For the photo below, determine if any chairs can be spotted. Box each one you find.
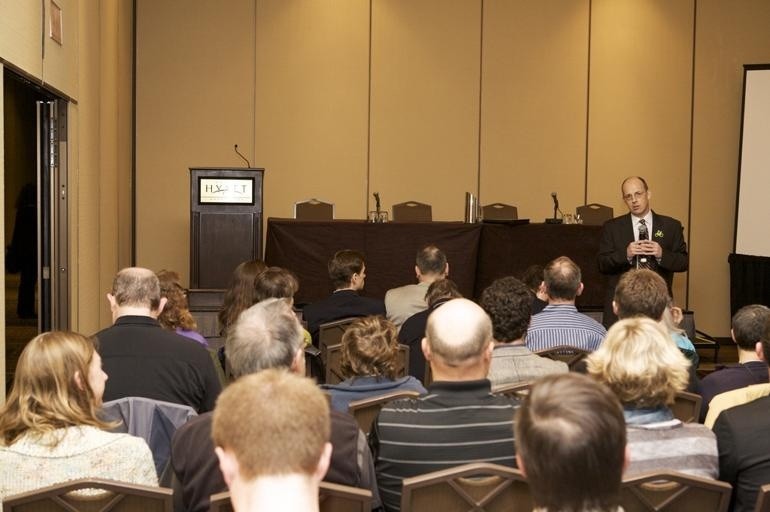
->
[349,390,422,440]
[619,470,732,510]
[319,482,372,512]
[532,345,591,365]
[301,317,432,390]
[210,491,232,510]
[491,381,535,400]
[481,201,519,220]
[292,196,334,219]
[401,461,533,509]
[8,478,175,512]
[752,484,770,511]
[392,199,434,220]
[670,391,703,422]
[574,202,613,224]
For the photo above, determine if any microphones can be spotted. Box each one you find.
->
[373,191,380,211]
[234,144,250,168]
[639,225,648,263]
[551,192,559,207]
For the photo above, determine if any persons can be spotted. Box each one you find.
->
[599,176,689,330]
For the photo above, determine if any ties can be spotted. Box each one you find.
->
[637,218,651,272]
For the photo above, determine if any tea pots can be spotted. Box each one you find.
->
[464,191,485,223]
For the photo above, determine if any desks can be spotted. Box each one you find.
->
[265,216,612,308]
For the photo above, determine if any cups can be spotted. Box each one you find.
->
[377,211,388,225]
[573,213,584,226]
[367,212,378,223]
[561,211,573,224]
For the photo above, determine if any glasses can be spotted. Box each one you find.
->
[623,190,644,199]
